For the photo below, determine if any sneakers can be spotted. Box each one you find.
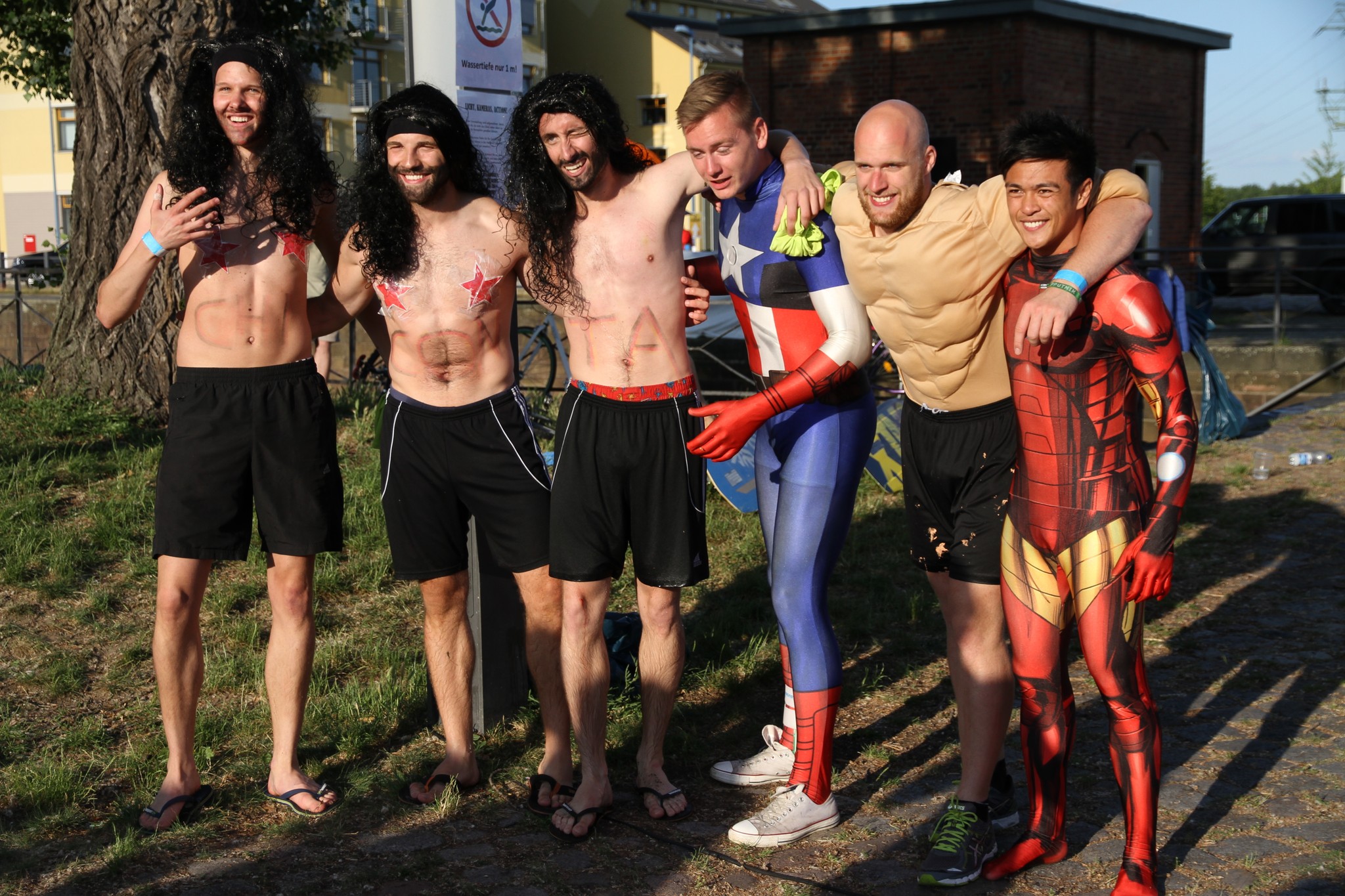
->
[913,788,997,885]
[927,757,1019,844]
[727,783,840,847]
[710,725,796,787]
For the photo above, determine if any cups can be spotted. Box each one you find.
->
[1253,452,1274,480]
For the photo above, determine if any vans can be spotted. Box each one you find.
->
[1198,194,1345,315]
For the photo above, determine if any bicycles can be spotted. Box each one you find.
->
[351,344,391,391]
[515,304,575,403]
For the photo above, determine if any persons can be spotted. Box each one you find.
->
[294,80,709,814]
[497,74,828,843]
[816,97,1152,888]
[96,38,390,835]
[673,68,880,846]
[995,116,1196,896]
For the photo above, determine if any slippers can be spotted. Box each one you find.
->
[397,773,482,805]
[139,784,214,836]
[263,781,343,817]
[548,802,614,843]
[527,773,576,815]
[638,786,693,821]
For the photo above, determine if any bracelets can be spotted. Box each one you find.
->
[1043,269,1088,305]
[141,231,167,257]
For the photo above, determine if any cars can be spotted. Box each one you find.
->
[11,241,74,287]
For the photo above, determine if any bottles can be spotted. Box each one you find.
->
[1289,450,1332,466]
